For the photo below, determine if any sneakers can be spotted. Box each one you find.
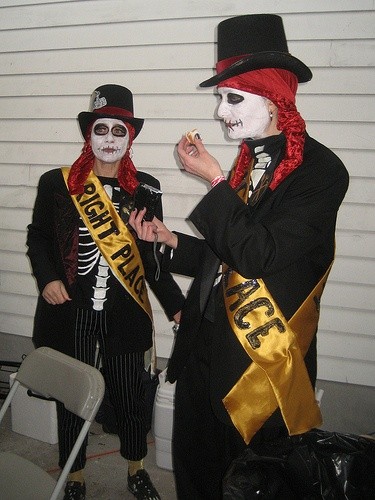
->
[126,467,160,500]
[62,480,86,500]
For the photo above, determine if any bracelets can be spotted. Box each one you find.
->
[171,321,180,333]
[210,175,227,188]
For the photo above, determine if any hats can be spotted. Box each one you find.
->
[199,14,313,87]
[78,84,145,141]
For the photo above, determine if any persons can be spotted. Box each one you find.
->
[128,13,350,500]
[24,85,188,500]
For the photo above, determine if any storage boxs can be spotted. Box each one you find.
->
[10,373,58,444]
[154,369,178,472]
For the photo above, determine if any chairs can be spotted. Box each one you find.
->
[0,347,106,500]
